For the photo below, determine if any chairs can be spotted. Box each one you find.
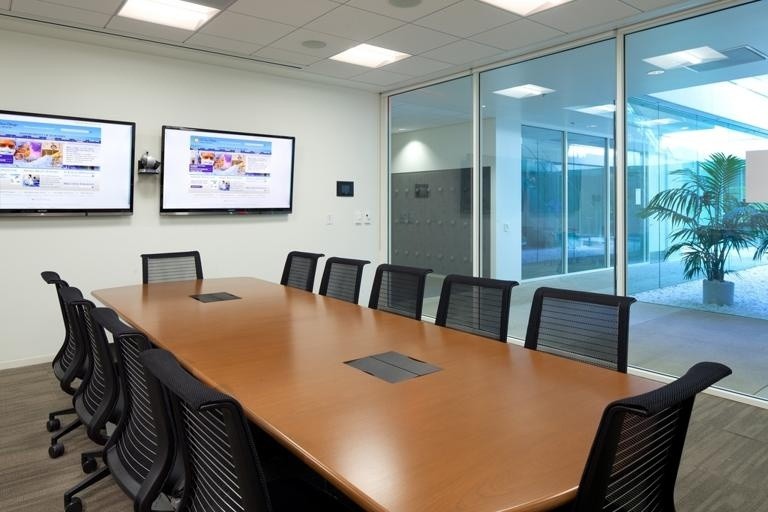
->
[524,287,637,374]
[140,249,206,284]
[62,309,186,512]
[321,256,370,304]
[129,350,275,512]
[281,249,323,293]
[57,285,111,471]
[369,263,432,321]
[33,271,88,458]
[431,276,520,340]
[574,360,735,511]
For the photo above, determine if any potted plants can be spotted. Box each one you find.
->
[640,152,767,306]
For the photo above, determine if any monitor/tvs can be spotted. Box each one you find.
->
[0,111,136,216]
[160,125,295,217]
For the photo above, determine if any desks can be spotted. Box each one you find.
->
[91,277,674,512]
